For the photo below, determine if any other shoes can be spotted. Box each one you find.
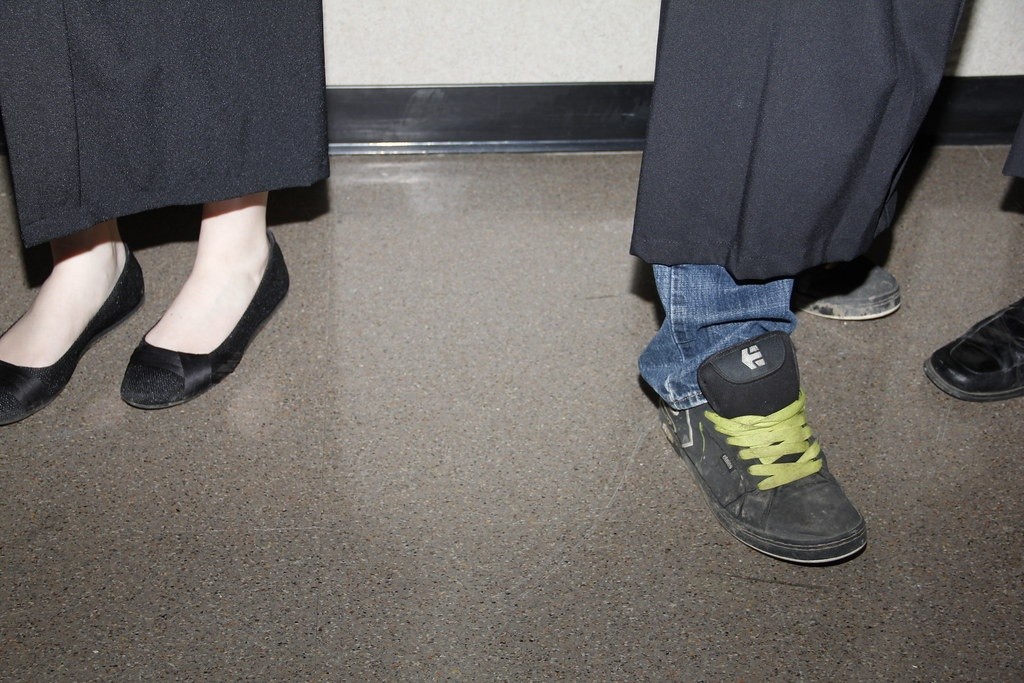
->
[0,242,145,423]
[121,230,288,408]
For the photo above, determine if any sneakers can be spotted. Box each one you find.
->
[792,255,901,319]
[660,331,868,564]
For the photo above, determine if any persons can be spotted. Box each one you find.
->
[625,1,971,566]
[0,0,331,429]
[920,293,1024,403]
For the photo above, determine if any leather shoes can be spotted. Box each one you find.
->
[924,294,1024,400]
